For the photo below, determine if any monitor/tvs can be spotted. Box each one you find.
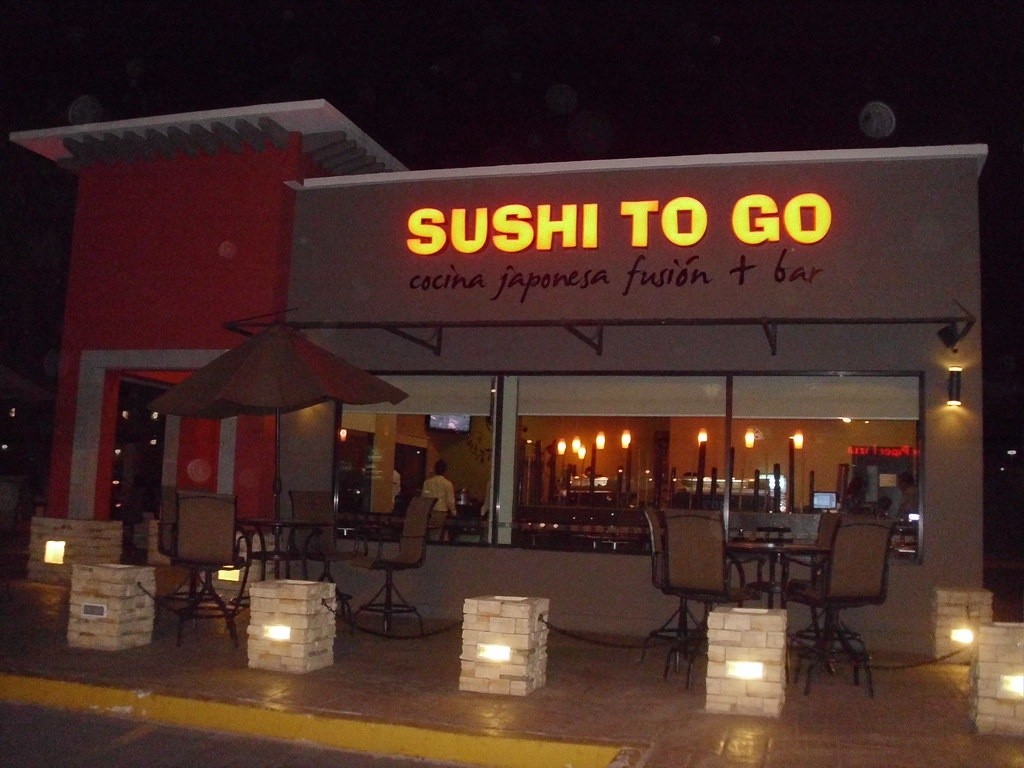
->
[425,415,472,434]
[813,492,837,509]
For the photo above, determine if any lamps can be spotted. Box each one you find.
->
[556,428,804,461]
[946,367,963,407]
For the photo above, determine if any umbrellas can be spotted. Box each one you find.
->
[145,321,408,521]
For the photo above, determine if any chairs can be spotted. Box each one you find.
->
[349,495,440,637]
[157,486,243,648]
[787,511,862,661]
[640,511,680,662]
[284,490,353,619]
[791,522,891,701]
[646,515,755,689]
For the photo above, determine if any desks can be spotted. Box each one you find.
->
[235,519,355,626]
[718,538,832,607]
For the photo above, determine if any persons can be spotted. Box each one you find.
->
[344,458,371,522]
[421,459,457,516]
[841,476,868,511]
[897,471,919,515]
